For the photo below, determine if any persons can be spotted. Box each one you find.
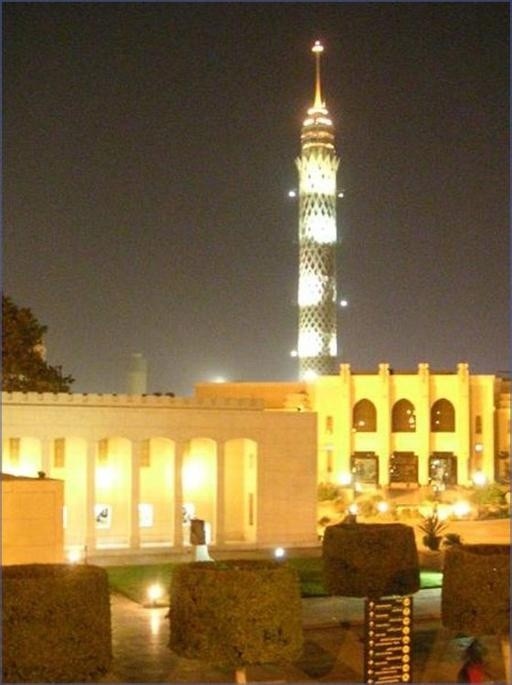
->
[456,640,496,685]
